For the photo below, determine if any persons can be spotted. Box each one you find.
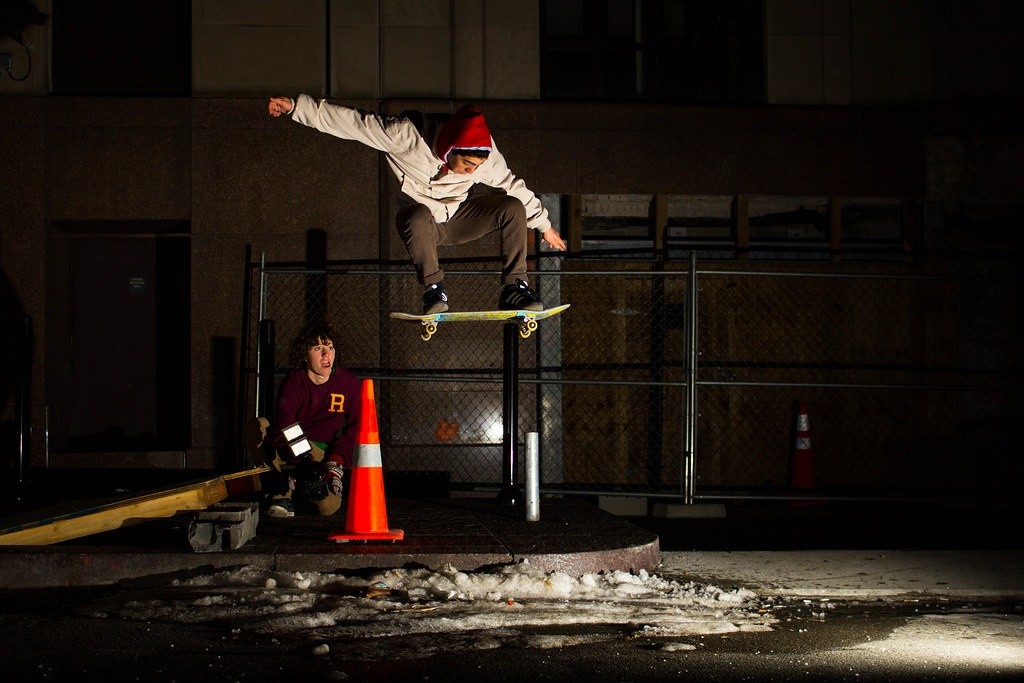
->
[244,321,361,518]
[266,92,568,317]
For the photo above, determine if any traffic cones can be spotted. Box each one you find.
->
[333,379,405,545]
[786,405,826,506]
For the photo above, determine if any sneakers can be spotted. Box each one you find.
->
[497,278,543,311]
[423,281,449,315]
[267,498,295,518]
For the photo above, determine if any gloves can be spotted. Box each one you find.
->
[273,473,297,499]
[323,461,344,495]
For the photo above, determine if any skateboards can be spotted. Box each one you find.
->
[388,301,572,342]
